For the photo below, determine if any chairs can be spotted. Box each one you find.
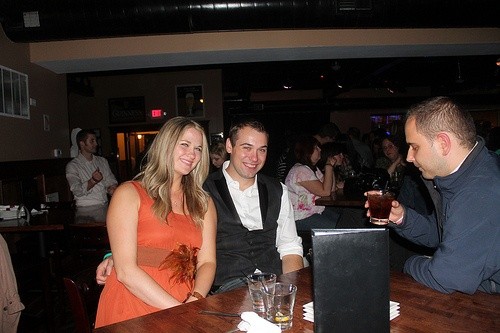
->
[61,265,105,333]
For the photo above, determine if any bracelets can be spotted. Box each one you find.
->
[394,211,404,225]
[92,176,98,182]
[104,253,112,260]
[326,163,333,167]
[187,292,205,301]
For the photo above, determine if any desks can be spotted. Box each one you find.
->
[316,188,396,206]
[93,263,500,333]
[0,204,108,333]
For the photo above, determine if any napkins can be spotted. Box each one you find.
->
[303,300,401,323]
[237,311,282,333]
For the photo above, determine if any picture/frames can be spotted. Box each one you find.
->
[174,84,205,119]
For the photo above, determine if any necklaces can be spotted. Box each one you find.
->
[171,191,183,206]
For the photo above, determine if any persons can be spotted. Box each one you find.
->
[209,132,230,169]
[65,130,119,207]
[364,96,500,295]
[134,139,153,173]
[259,121,435,255]
[93,116,218,328]
[97,119,303,296]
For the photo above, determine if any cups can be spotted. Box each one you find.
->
[261,283,297,330]
[367,190,395,226]
[247,273,277,311]
[48,148,61,158]
